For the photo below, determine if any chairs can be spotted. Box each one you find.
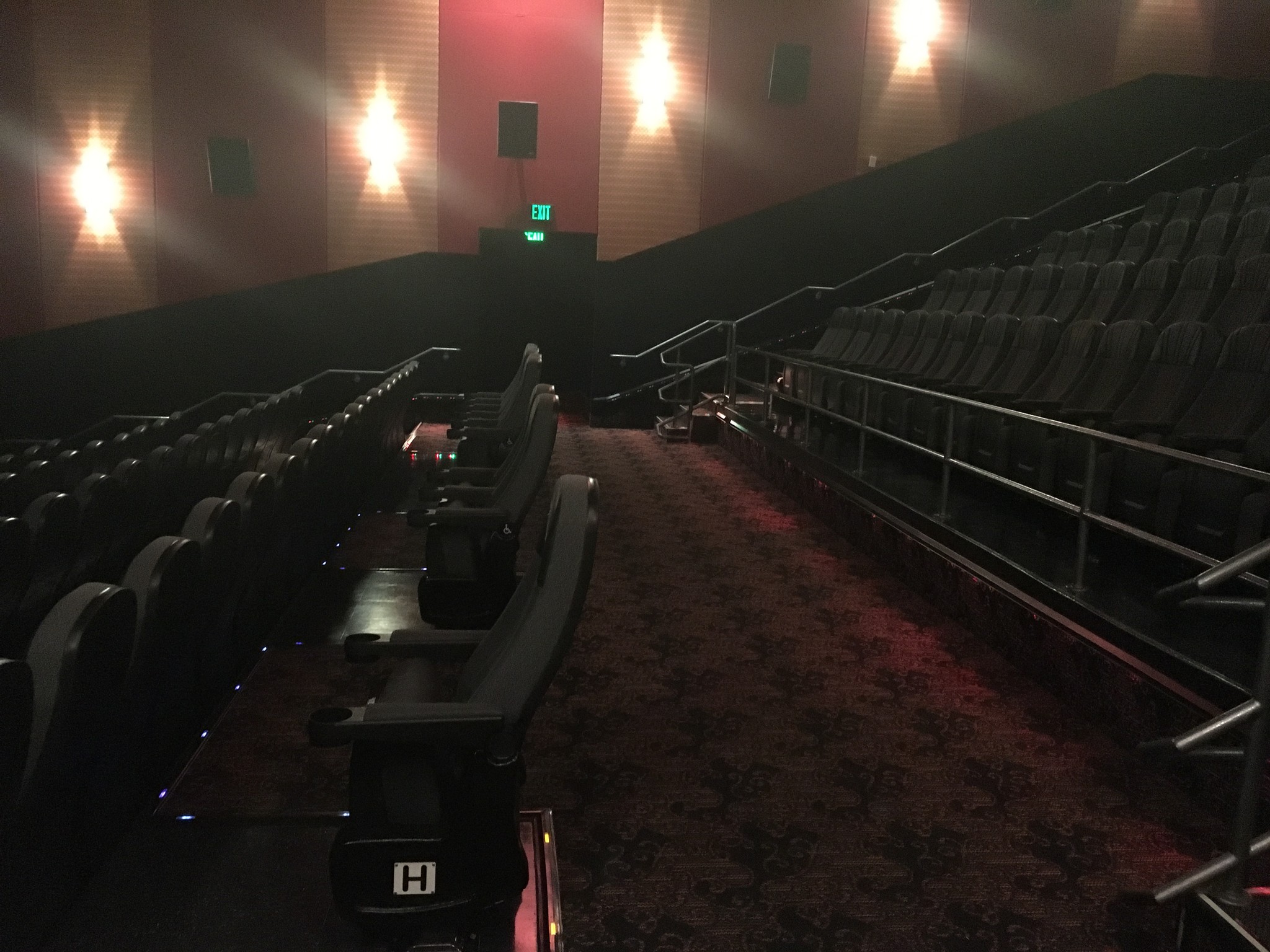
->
[0,342,598,952]
[777,153,1270,638]
